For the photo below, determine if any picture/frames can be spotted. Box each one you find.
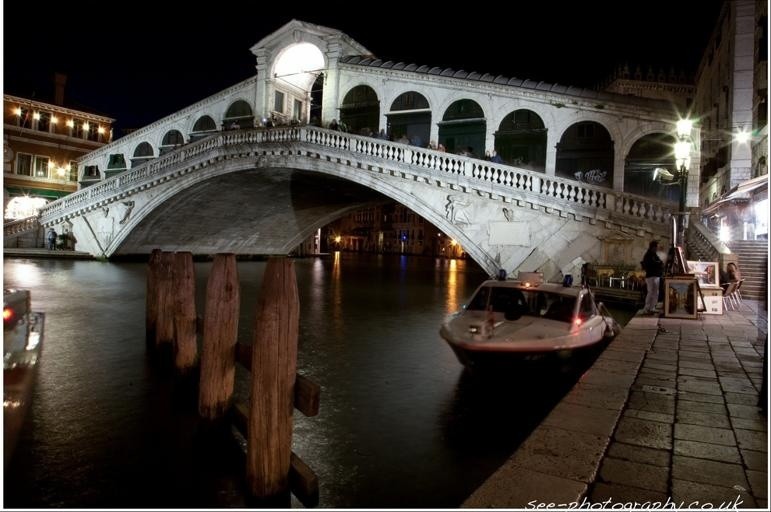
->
[662,276,697,321]
[685,259,721,287]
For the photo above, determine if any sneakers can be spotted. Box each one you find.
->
[641,307,660,316]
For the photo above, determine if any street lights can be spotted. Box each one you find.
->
[674,115,698,256]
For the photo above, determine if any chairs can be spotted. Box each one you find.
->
[720,278,745,312]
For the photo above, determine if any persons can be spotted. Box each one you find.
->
[368,127,504,165]
[641,240,666,316]
[337,120,348,133]
[230,115,307,131]
[46,227,71,251]
[328,118,339,129]
[117,199,136,224]
[720,262,740,294]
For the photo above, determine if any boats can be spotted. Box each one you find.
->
[2,284,50,460]
[437,267,623,375]
